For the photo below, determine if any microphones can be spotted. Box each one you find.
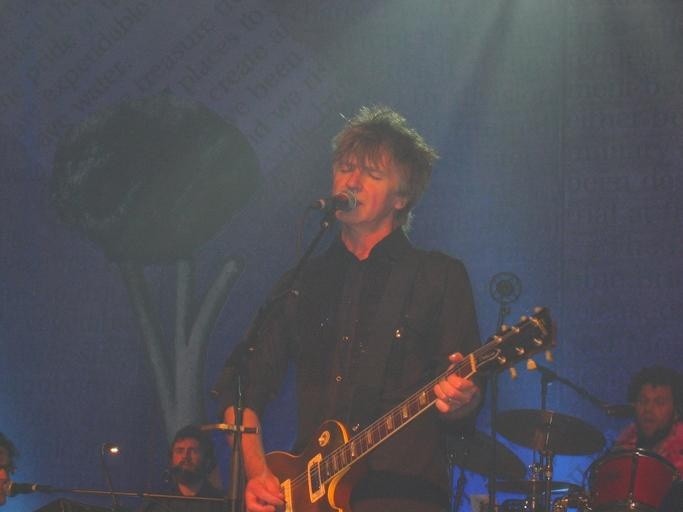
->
[490,273,522,304]
[310,190,356,212]
[3,481,50,497]
[605,404,636,416]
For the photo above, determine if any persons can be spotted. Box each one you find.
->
[588,364,683,512]
[0,432,20,506]
[143,425,230,512]
[218,105,489,511]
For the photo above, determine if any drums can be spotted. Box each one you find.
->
[585,449,677,510]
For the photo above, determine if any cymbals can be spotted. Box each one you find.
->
[482,478,572,491]
[493,411,608,456]
[454,429,528,480]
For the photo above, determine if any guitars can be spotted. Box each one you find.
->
[262,305,556,512]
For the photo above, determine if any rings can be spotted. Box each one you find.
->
[448,398,451,403]
